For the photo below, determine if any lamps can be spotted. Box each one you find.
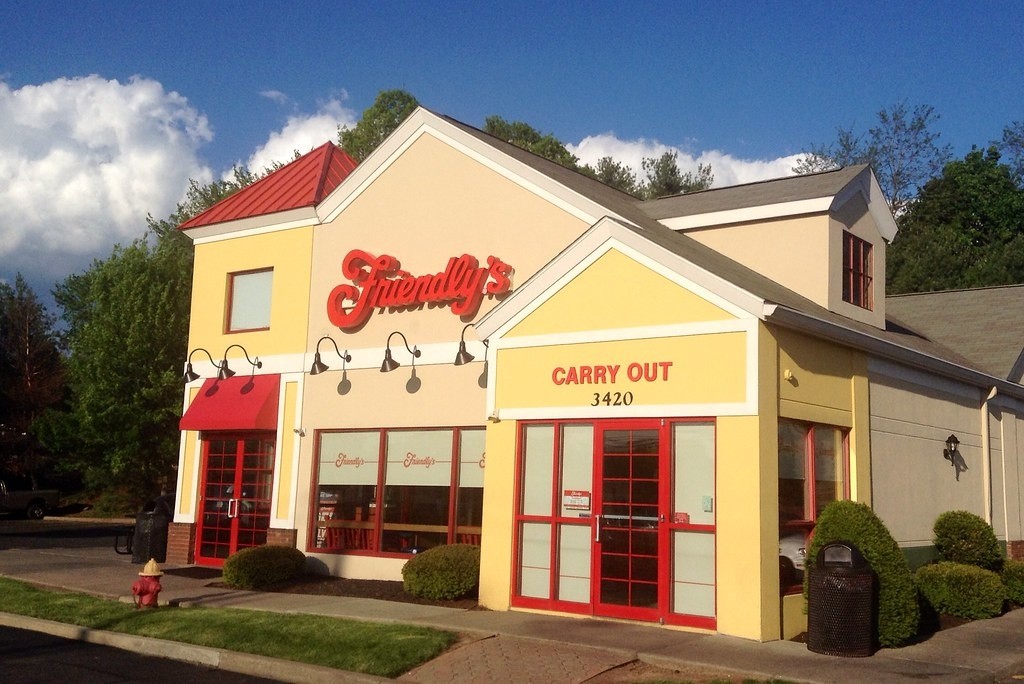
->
[182,348,222,383]
[454,323,489,366]
[943,434,960,466]
[219,344,262,380]
[380,331,421,372]
[309,337,352,375]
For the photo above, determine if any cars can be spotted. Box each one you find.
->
[779,512,806,592]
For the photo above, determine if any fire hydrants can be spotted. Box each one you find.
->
[133,558,164,608]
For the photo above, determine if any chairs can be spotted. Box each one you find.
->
[324,515,478,550]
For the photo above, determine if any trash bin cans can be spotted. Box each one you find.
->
[131,503,170,565]
[807,541,878,659]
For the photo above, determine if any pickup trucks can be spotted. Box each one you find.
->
[0,480,60,521]
